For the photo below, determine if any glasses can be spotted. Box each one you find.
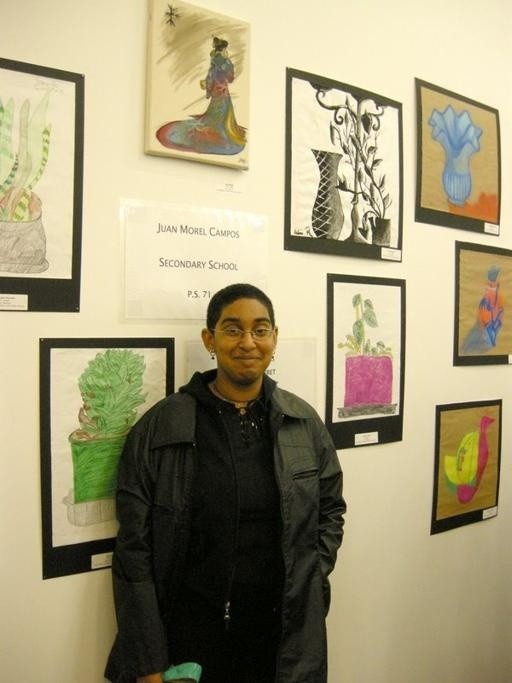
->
[209,328,272,341]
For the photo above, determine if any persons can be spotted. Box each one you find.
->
[104,285,347,683]
[156,37,247,156]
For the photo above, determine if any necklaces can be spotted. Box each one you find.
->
[212,383,257,415]
